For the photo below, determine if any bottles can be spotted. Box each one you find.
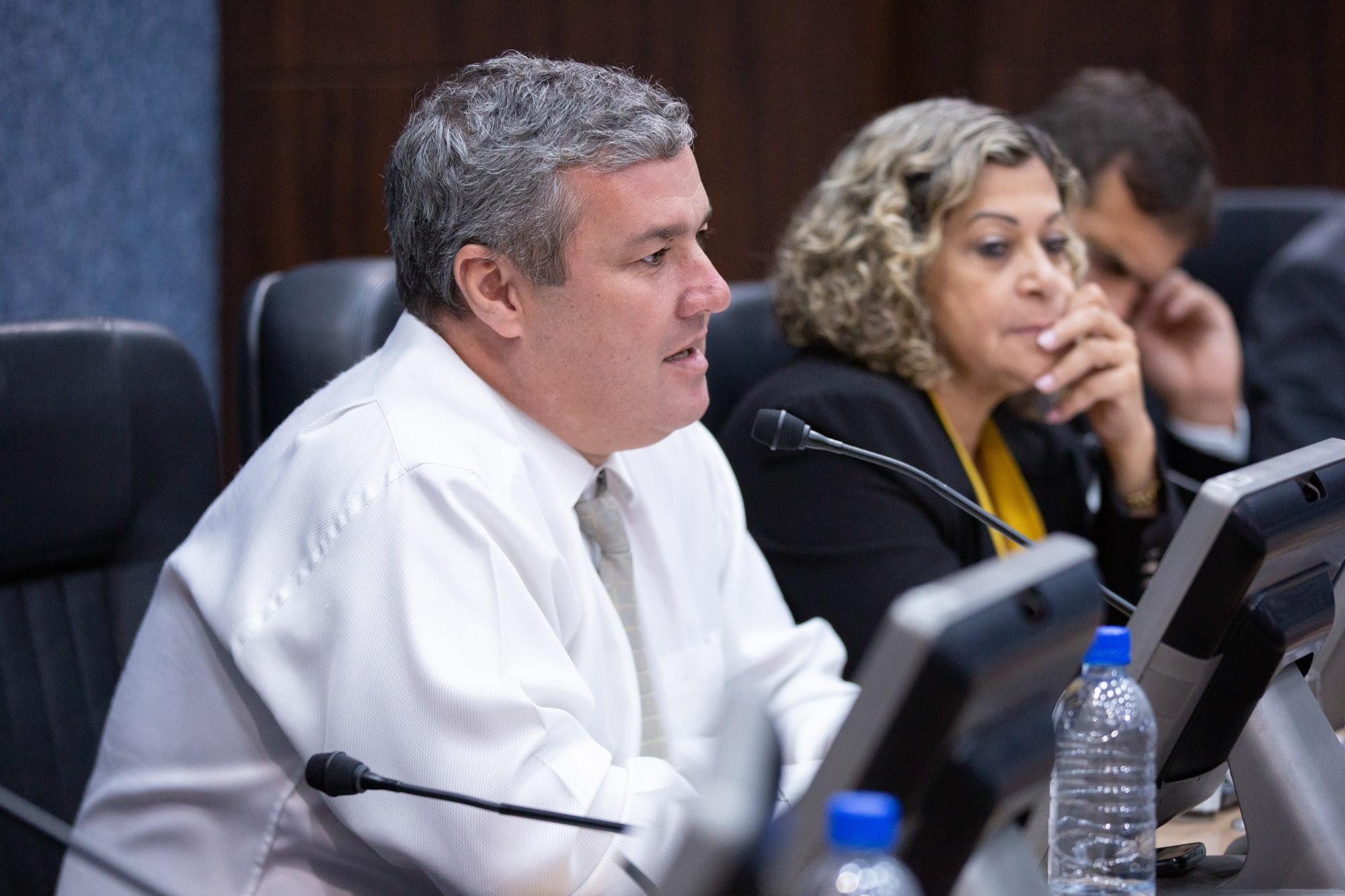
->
[794,789,926,896]
[1045,625,1160,896]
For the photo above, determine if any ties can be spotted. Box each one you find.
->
[574,469,666,759]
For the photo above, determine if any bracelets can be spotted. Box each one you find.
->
[1122,479,1157,509]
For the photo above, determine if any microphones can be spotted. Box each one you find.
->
[751,409,1136,616]
[304,753,635,837]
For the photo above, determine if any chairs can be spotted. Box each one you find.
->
[0,318,224,896]
[239,253,405,470]
[698,279,800,447]
[1173,185,1344,328]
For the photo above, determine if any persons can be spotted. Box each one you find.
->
[717,98,1182,683]
[57,53,862,896]
[992,69,1322,573]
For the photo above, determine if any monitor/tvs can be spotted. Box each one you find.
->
[767,438,1345,896]
